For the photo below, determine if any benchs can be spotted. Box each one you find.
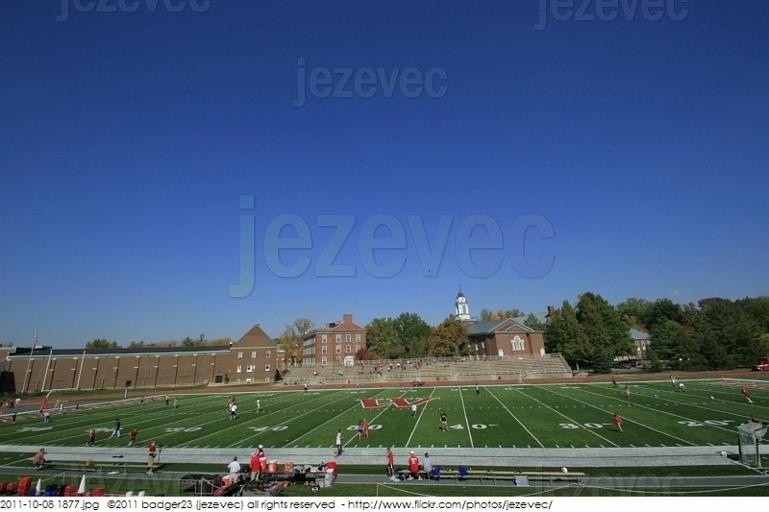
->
[397,469,586,488]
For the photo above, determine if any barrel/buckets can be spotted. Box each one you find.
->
[284,461,292,472]
[458,465,468,476]
[432,467,440,480]
[268,459,279,472]
[259,456,268,471]
[222,475,230,485]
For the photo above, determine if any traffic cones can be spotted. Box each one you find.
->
[33,478,42,495]
[76,474,86,494]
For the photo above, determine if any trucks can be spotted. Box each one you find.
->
[751,357,769,372]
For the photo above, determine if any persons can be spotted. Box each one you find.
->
[0,351,754,497]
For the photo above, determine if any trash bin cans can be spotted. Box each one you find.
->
[45,484,58,496]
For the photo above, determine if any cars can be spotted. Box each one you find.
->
[612,359,640,369]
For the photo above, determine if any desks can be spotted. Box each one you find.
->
[178,468,327,497]
